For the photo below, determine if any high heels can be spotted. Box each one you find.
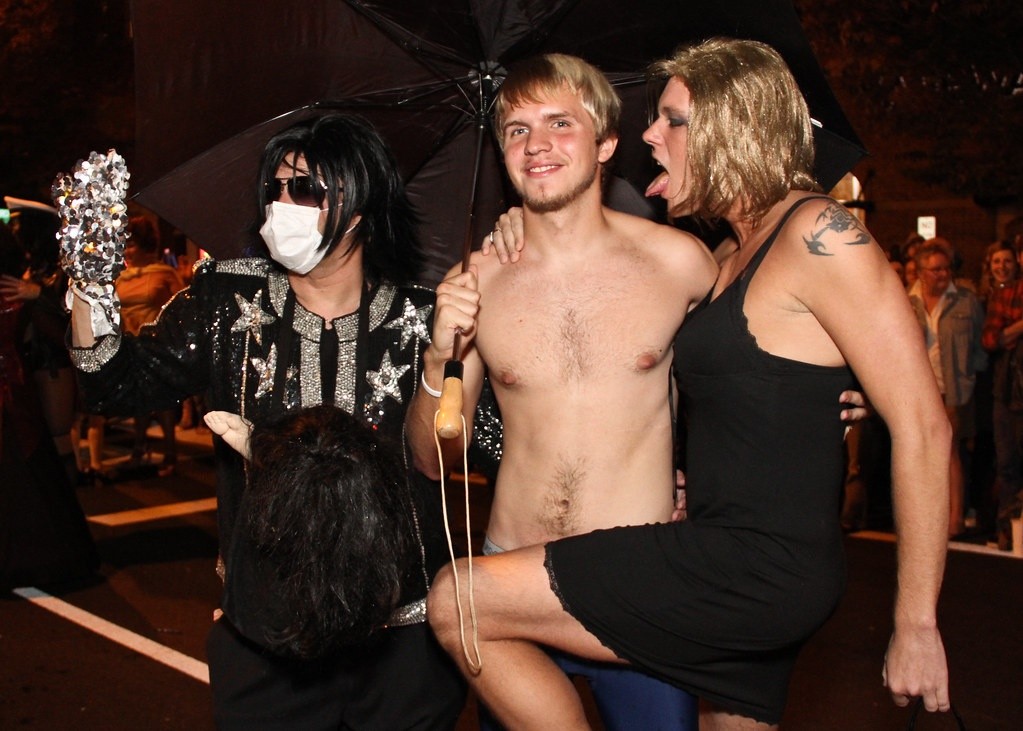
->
[156,455,178,476]
[127,439,152,466]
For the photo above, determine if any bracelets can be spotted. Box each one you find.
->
[421,371,442,398]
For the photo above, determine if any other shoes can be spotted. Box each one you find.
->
[999,532,1013,551]
[89,469,109,486]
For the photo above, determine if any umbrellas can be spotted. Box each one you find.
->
[128,1,846,434]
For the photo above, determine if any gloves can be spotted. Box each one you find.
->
[52,149,130,336]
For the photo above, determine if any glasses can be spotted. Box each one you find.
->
[261,177,344,207]
[920,266,950,274]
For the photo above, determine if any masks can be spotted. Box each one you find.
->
[260,200,348,276]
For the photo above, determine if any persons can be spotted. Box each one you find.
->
[3,201,213,483]
[422,39,956,731]
[54,117,438,731]
[407,54,874,731]
[853,215,1023,553]
